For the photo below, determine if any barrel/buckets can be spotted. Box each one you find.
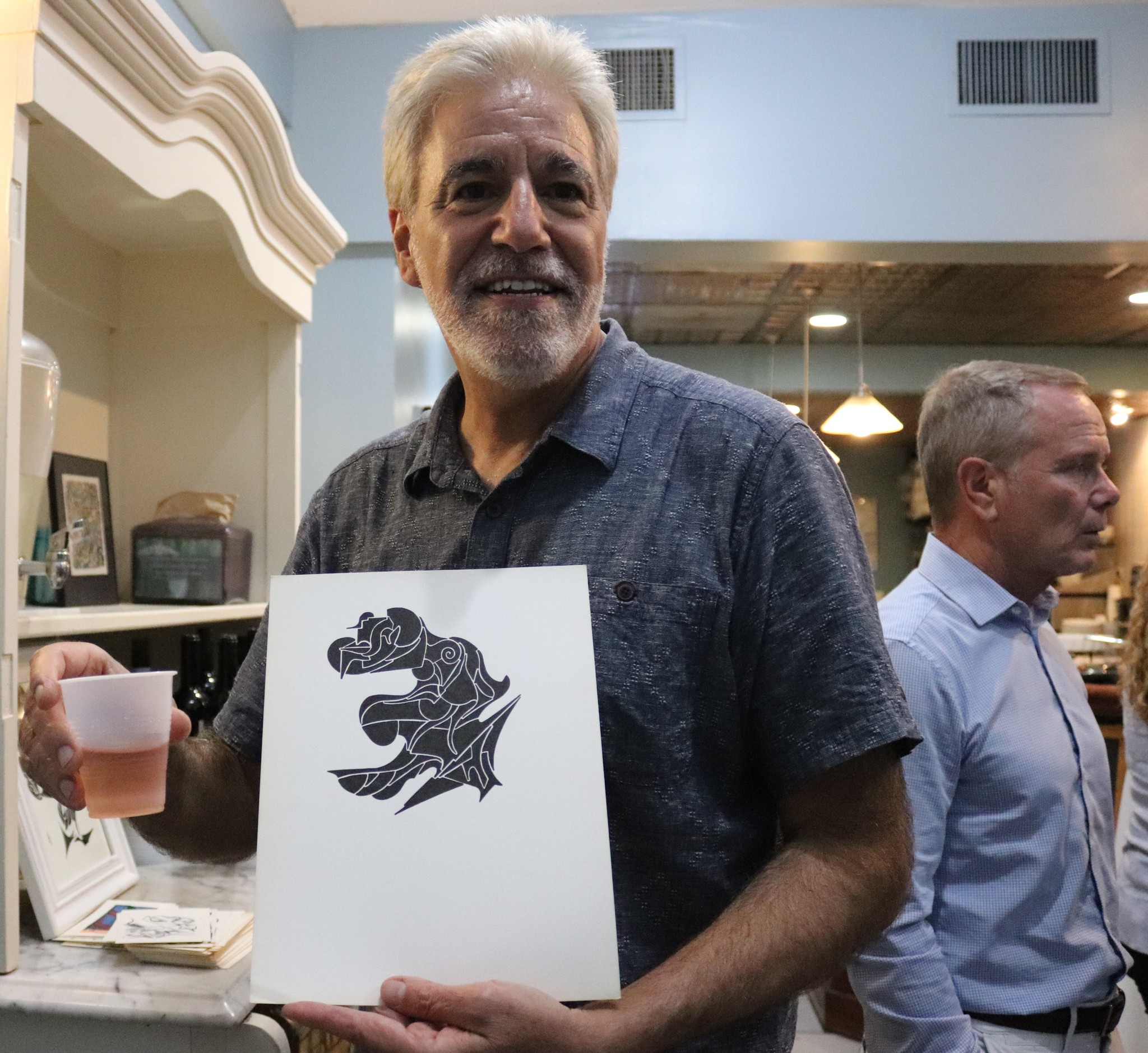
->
[18,328,84,608]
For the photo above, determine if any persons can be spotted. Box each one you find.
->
[19,18,925,1053]
[1113,563,1148,1015]
[844,361,1134,1053]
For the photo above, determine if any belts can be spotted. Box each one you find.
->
[962,982,1126,1034]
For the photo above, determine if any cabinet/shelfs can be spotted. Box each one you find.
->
[0,1,370,1053]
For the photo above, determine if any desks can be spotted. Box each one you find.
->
[1071,644,1132,828]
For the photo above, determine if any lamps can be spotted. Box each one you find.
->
[772,259,911,470]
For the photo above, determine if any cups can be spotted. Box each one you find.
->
[57,670,178,820]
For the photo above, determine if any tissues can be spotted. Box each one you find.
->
[125,487,256,606]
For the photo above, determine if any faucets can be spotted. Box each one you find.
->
[14,514,85,591]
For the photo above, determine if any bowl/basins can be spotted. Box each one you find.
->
[1080,664,1119,685]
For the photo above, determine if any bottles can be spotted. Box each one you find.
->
[32,527,59,605]
[129,627,257,738]
